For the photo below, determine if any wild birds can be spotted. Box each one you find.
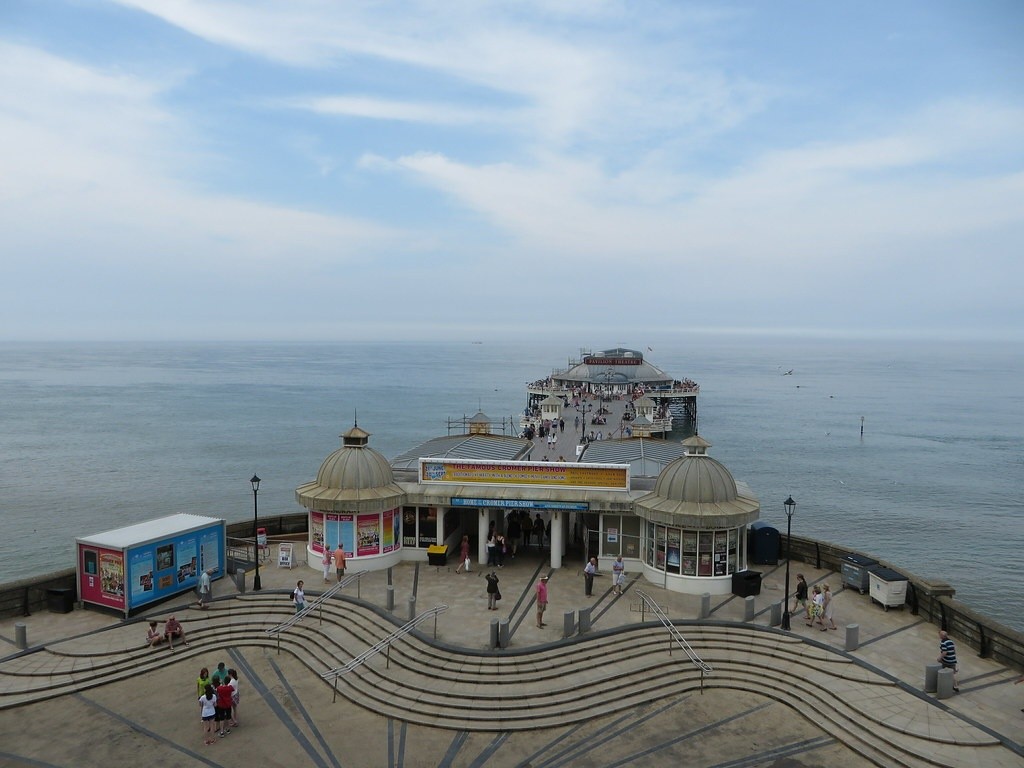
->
[824,431,831,437]
[783,368,794,375]
[648,345,653,351]
[493,388,498,391]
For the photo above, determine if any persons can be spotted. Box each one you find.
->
[592,413,606,425]
[322,544,347,585]
[524,401,564,449]
[620,406,637,437]
[197,662,239,745]
[613,555,624,594]
[164,615,189,651]
[584,558,596,598]
[542,456,549,461]
[456,536,473,574]
[789,574,837,631]
[293,580,305,621]
[197,569,213,609]
[485,573,501,610]
[574,416,586,434]
[487,527,505,569]
[654,405,669,421]
[489,508,560,558]
[556,456,566,462]
[528,377,697,407]
[590,430,613,441]
[937,631,960,692]
[536,576,549,629]
[146,620,163,648]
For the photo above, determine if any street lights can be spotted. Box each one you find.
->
[250,471,262,591]
[779,494,798,630]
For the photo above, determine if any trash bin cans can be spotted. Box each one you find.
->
[47,588,73,612]
[732,570,761,597]
[868,568,908,612]
[841,554,879,595]
[428,545,448,565]
[257,528,266,548]
[749,521,781,565]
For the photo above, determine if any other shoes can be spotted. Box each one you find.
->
[952,686,959,693]
[537,624,542,628]
[620,591,622,594]
[587,594,590,598]
[456,570,460,574]
[204,739,208,745]
[171,647,174,651]
[803,616,836,631]
[211,738,215,745]
[224,728,230,733]
[184,641,189,646]
[217,731,224,737]
[539,622,547,627]
[614,591,617,595]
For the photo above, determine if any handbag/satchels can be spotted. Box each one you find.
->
[465,557,471,571]
[617,570,626,585]
[494,590,501,600]
[290,590,296,599]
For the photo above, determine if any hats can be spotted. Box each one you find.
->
[539,575,548,579]
